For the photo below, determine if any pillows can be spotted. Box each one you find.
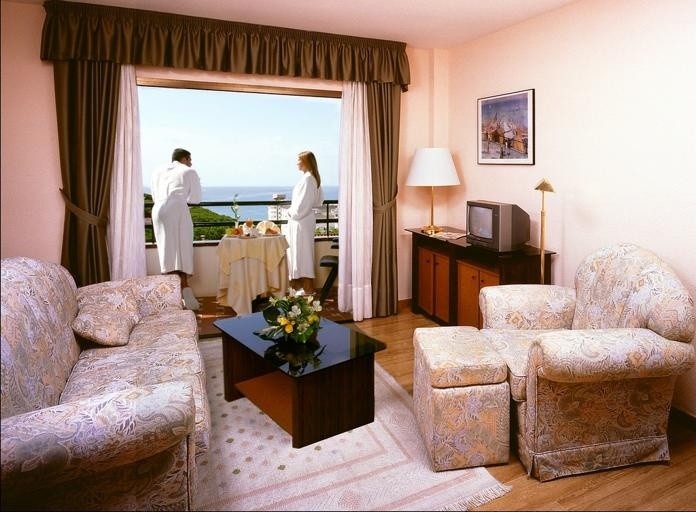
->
[70,283,142,347]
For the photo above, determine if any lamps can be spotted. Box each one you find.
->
[404,147,461,235]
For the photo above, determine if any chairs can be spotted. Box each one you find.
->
[477,241,696,482]
[319,237,339,306]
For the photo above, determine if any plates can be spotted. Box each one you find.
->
[226,231,281,239]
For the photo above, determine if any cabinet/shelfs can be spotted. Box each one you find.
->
[411,232,551,331]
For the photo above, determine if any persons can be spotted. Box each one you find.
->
[151,148,202,311]
[283,150,324,295]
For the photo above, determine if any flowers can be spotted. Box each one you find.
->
[230,193,241,222]
[250,286,324,343]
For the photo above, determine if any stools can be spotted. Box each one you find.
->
[412,325,511,473]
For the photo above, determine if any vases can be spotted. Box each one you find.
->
[307,331,318,344]
[234,221,240,229]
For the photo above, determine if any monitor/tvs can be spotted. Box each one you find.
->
[467,200,529,253]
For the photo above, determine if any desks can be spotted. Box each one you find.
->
[216,233,291,316]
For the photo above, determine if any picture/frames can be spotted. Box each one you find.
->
[476,88,535,165]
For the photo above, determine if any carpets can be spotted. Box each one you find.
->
[184,285,355,336]
[194,333,513,512]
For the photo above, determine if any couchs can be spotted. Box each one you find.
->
[0,245,211,512]
